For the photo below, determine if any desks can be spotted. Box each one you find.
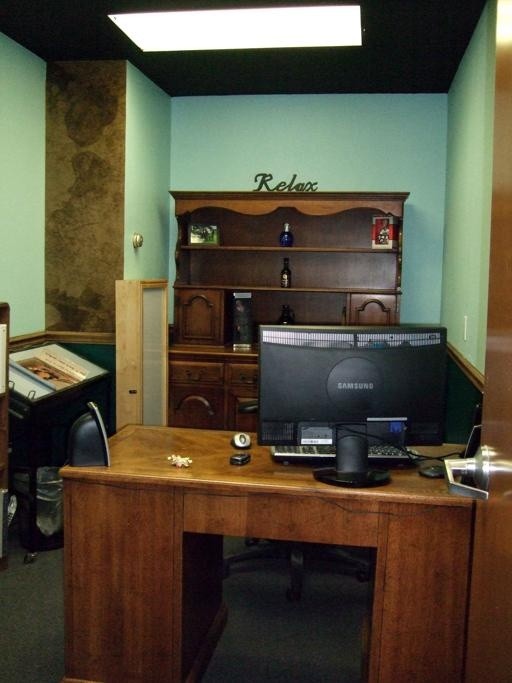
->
[60,425,477,683]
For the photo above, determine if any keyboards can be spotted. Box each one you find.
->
[270,442,410,465]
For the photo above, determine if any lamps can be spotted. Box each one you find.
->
[29,402,110,492]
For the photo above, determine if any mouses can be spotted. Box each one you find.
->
[232,433,251,449]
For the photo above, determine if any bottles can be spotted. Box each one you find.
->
[279,223,294,247]
[278,303,295,324]
[280,257,291,288]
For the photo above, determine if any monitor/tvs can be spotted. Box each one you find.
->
[259,324,447,488]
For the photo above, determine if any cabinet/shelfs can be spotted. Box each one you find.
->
[169,191,410,432]
